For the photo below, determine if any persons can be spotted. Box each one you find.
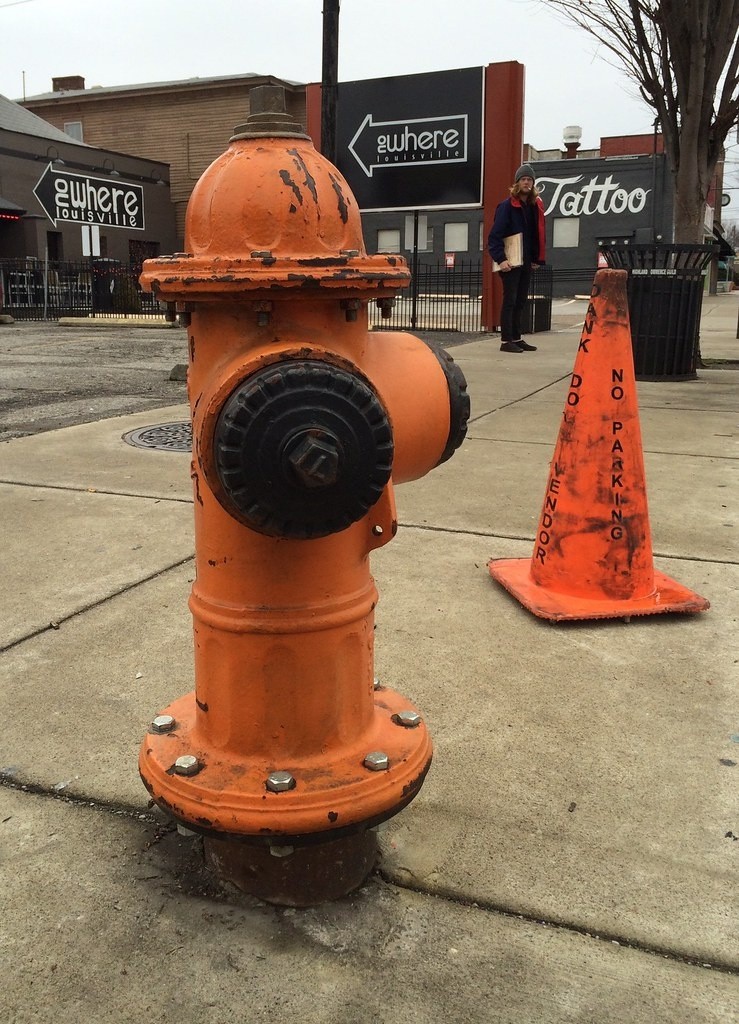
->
[487,164,547,353]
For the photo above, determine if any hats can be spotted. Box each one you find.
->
[515,165,535,185]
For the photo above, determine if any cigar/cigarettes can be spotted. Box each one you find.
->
[528,188,531,190]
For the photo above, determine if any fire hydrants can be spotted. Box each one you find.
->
[136,82,471,906]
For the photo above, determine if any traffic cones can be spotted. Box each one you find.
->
[487,267,711,628]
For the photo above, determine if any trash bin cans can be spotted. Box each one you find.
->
[598,242,719,383]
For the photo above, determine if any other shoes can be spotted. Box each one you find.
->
[513,340,537,351]
[500,341,523,353]
